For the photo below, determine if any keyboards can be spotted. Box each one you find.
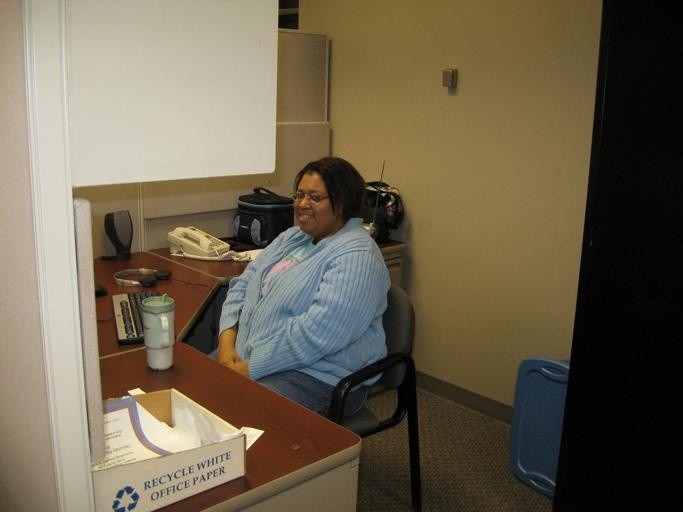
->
[112,290,176,345]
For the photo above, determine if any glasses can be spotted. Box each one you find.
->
[290,191,329,203]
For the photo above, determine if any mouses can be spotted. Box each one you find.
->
[95,285,108,297]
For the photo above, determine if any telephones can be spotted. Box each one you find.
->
[167,226,231,261]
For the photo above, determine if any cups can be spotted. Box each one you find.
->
[142,296,175,370]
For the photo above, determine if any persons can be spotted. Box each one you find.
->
[204,156,393,420]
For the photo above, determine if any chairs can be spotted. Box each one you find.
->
[324,282,422,512]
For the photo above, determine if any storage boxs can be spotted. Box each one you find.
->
[509,356,570,498]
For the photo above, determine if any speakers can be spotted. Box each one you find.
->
[100,210,133,261]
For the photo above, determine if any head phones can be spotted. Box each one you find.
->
[112,267,171,287]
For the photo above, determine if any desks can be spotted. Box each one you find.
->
[92,236,406,512]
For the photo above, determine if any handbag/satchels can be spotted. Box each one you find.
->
[233,186,294,249]
